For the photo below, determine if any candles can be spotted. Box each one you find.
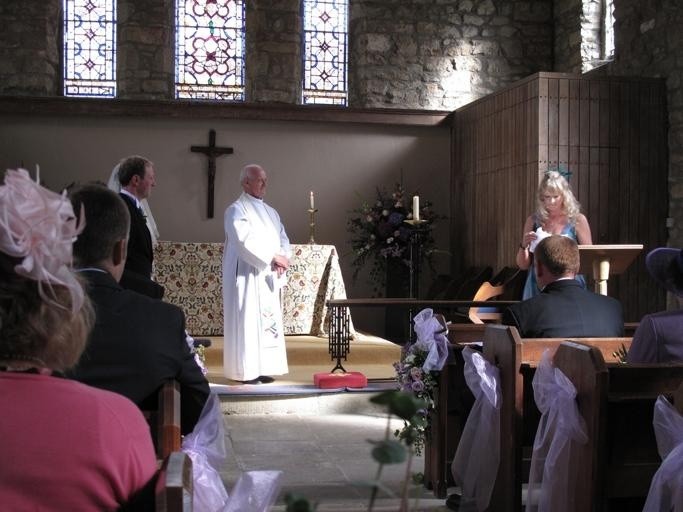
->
[310,191,314,210]
[413,195,420,220]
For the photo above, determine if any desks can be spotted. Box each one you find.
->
[153,241,356,336]
[325,298,523,373]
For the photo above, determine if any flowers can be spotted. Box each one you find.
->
[393,347,437,453]
[343,174,445,298]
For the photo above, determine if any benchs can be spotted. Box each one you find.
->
[424,322,682,512]
[139,382,195,512]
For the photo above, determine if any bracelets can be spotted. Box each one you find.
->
[520,243,526,250]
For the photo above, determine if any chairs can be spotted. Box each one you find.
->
[426,264,491,321]
[453,267,526,323]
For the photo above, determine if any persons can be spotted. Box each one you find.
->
[219,161,294,385]
[113,158,165,303]
[1,160,172,512]
[605,229,683,462]
[514,168,593,305]
[19,184,210,462]
[501,233,625,455]
[107,155,163,284]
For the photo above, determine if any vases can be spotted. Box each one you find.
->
[385,247,418,342]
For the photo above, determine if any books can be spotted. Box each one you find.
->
[266,269,289,292]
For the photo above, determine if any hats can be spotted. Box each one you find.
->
[646,248,681,299]
